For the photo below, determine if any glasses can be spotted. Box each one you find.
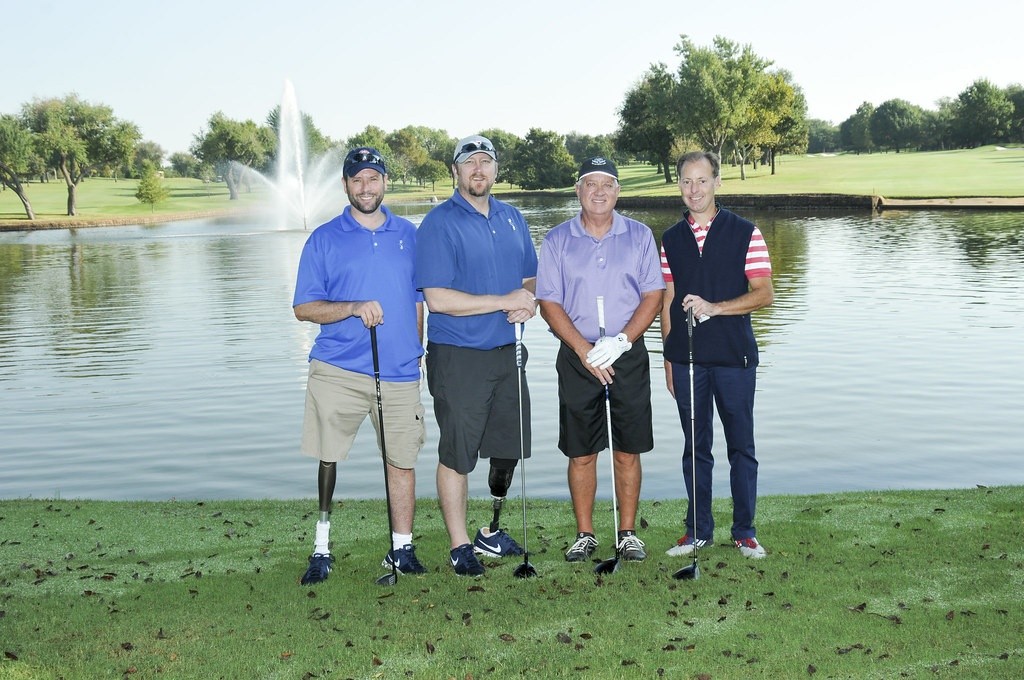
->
[454,141,497,164]
[344,152,385,174]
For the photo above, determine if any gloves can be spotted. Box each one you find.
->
[586,333,632,370]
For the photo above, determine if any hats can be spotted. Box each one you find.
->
[578,156,619,182]
[454,135,496,163]
[343,147,386,177]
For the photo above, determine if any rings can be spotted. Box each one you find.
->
[533,298,536,300]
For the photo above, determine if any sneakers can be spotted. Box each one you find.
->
[474,527,523,558]
[450,542,485,578]
[381,544,428,576]
[301,553,335,586]
[665,535,714,557]
[732,537,766,560]
[618,535,646,562]
[565,531,598,562]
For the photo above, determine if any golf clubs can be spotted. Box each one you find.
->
[512,322,538,578]
[594,294,623,575]
[369,325,399,587]
[669,300,702,580]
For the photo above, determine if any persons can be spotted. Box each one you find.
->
[534,156,667,563]
[292,148,424,588]
[660,152,775,560]
[414,134,540,578]
